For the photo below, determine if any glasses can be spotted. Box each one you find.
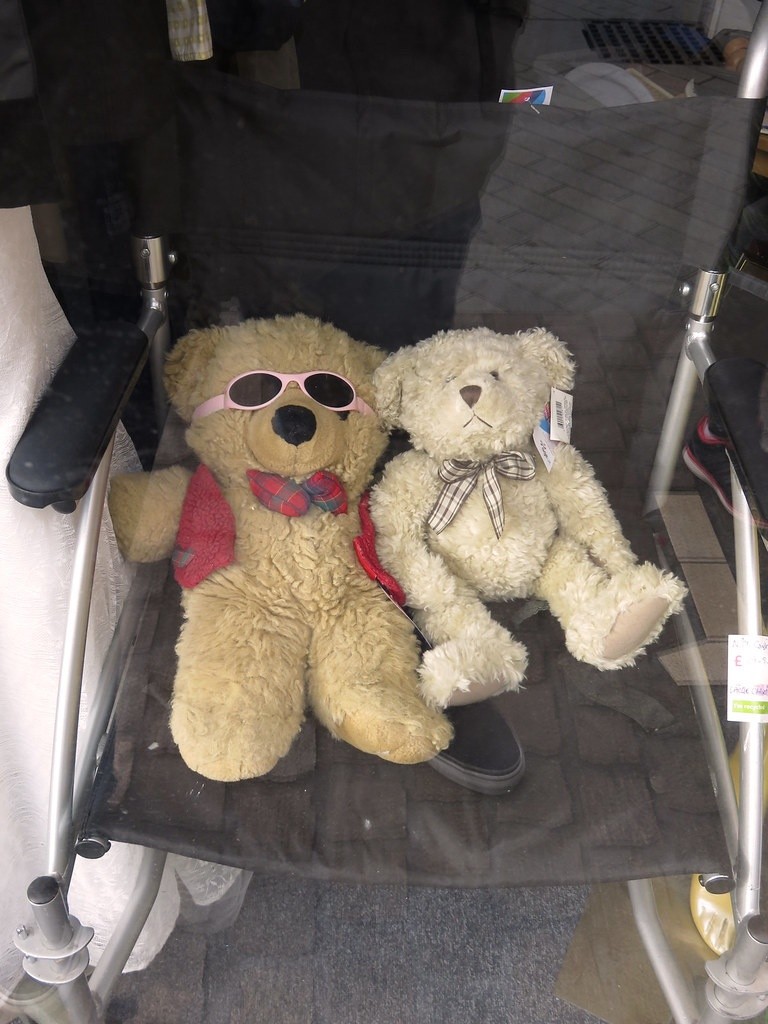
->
[189,371,376,423]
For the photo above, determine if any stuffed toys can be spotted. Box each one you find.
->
[368,325,691,712]
[109,310,458,784]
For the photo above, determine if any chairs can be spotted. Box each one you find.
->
[0,0,768,1024]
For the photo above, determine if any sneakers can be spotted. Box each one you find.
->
[427,699,524,794]
[684,417,768,536]
